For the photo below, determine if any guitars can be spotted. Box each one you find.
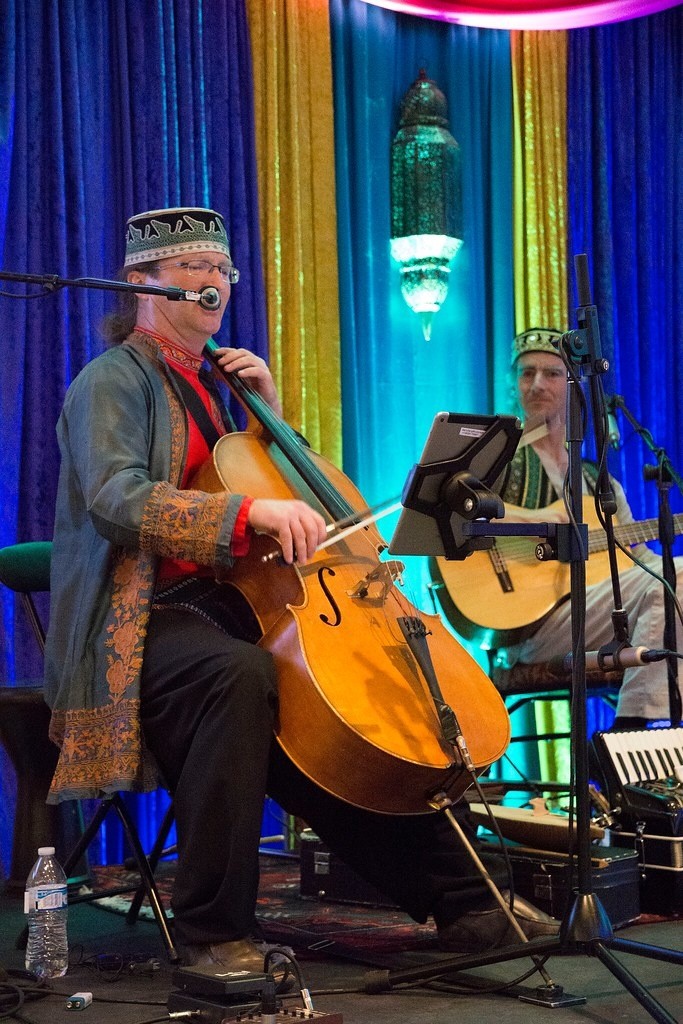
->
[428,492,683,651]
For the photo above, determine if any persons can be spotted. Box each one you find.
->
[40,205,575,984]
[471,328,682,805]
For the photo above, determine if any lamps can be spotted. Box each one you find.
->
[388,67,465,342]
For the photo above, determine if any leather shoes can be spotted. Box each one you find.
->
[172,939,296,995]
[433,890,563,954]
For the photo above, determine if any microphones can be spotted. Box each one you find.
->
[601,389,621,449]
[562,645,672,676]
[200,286,221,310]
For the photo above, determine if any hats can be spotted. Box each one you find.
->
[511,327,564,367]
[124,207,231,266]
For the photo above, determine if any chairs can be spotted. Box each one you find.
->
[424,555,623,812]
[1,538,183,967]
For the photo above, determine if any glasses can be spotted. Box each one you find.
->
[156,261,241,284]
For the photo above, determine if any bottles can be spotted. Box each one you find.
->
[24,847,69,979]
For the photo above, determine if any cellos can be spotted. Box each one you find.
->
[195,334,563,994]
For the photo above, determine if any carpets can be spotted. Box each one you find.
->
[90,850,443,963]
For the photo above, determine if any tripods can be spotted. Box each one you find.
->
[351,253,683,1023]
